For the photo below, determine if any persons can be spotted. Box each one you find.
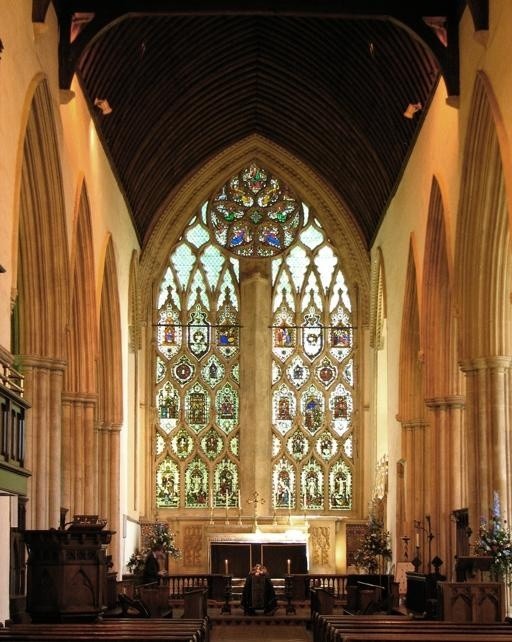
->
[140,542,168,589]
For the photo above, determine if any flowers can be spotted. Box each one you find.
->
[141,523,183,560]
[352,513,394,568]
[475,492,511,582]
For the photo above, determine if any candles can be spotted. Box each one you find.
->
[288,559,291,576]
[225,559,229,575]
[415,534,420,546]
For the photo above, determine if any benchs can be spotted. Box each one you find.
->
[311,611,512,642]
[0,616,211,642]
